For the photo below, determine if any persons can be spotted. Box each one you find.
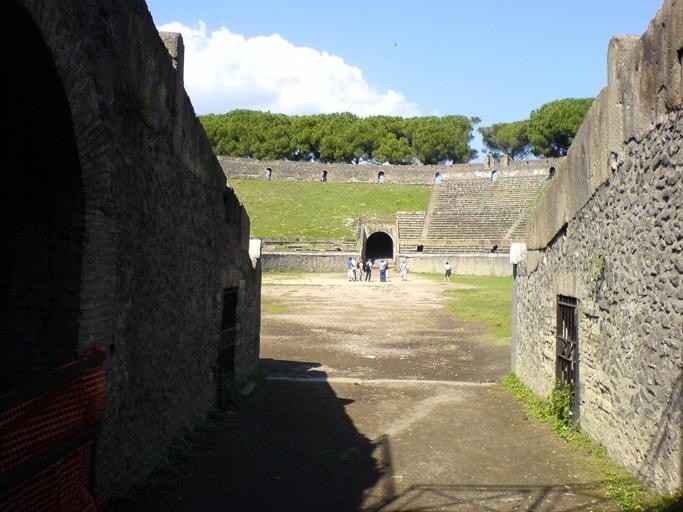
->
[358,260,363,281]
[399,259,408,281]
[351,256,357,280]
[364,258,372,282]
[346,257,355,282]
[441,262,451,281]
[378,257,388,283]
